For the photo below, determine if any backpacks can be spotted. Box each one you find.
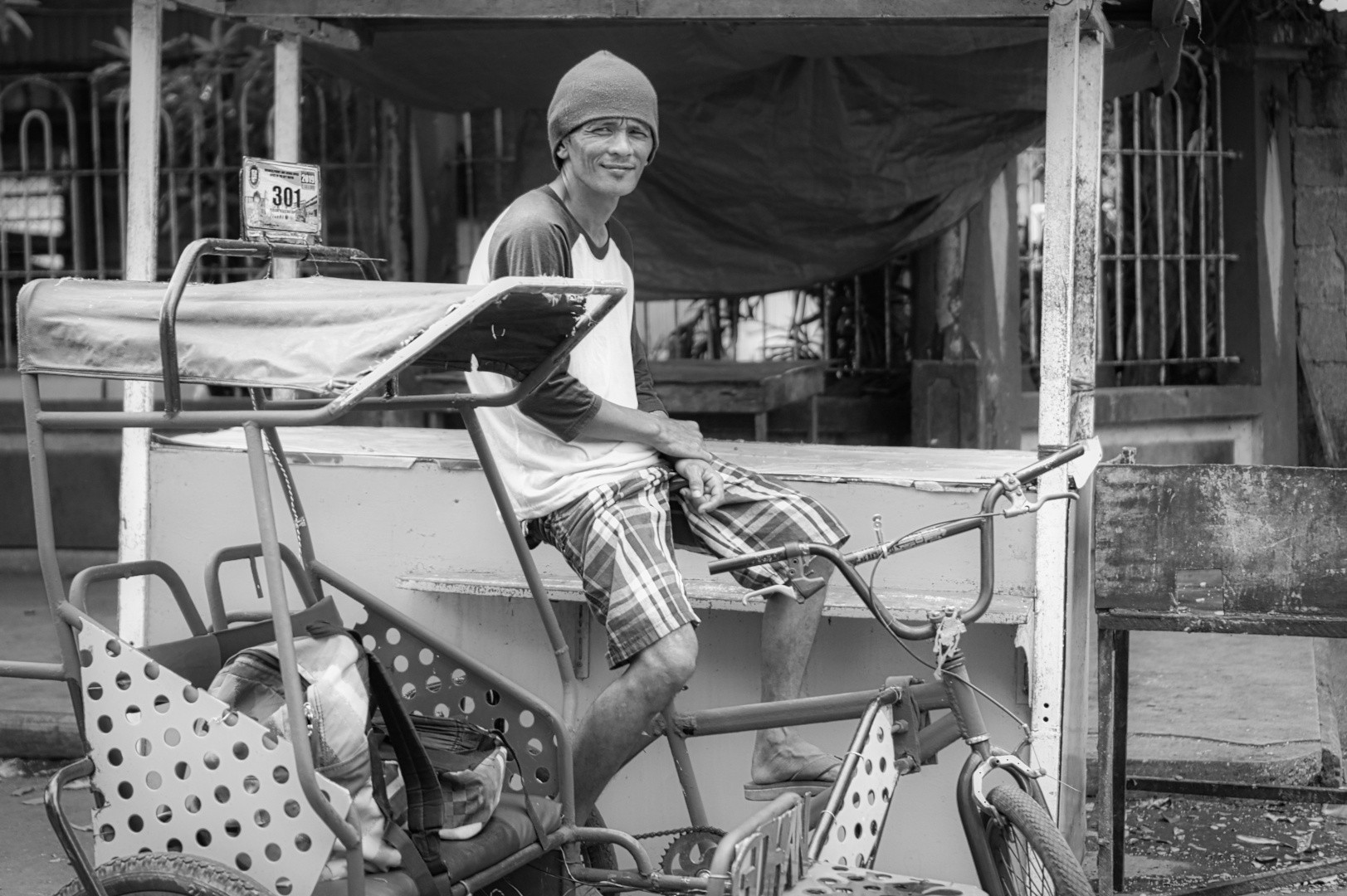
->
[210,618,454,880]
[370,710,550,852]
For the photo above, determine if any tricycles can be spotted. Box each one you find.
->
[12,237,1095,896]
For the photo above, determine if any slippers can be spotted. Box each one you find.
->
[743,751,845,800]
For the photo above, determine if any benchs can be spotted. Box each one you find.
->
[65,540,576,896]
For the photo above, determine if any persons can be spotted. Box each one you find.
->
[468,51,844,827]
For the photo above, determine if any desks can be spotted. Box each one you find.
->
[413,357,825,442]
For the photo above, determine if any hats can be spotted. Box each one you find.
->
[546,50,662,172]
